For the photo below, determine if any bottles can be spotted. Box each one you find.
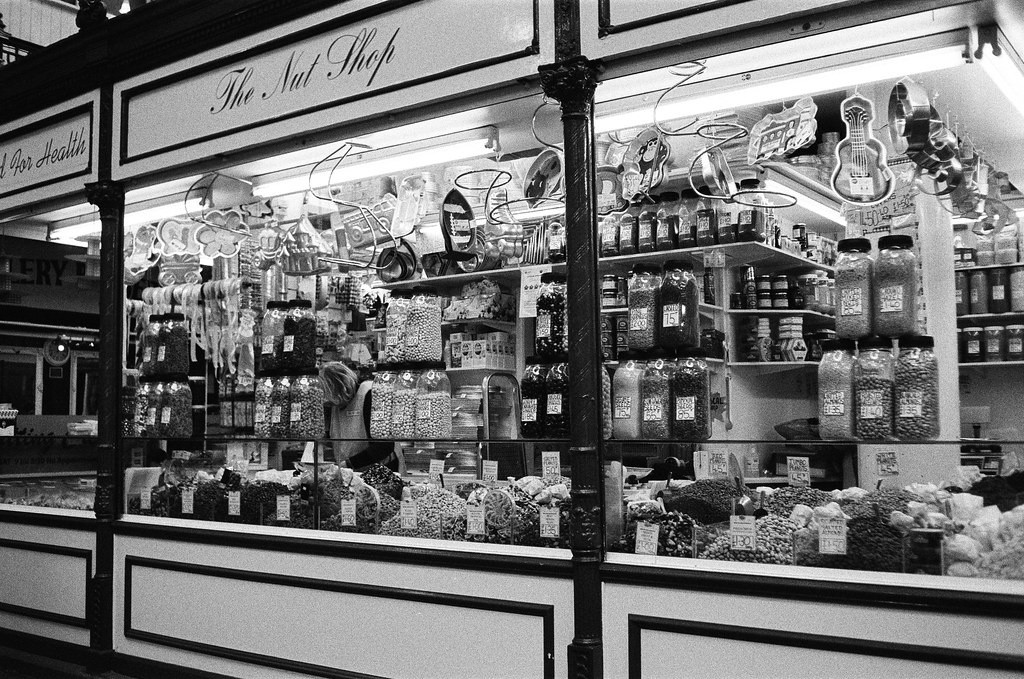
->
[746,446,760,478]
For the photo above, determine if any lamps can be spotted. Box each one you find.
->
[250,125,502,200]
[972,23,1024,115]
[589,26,978,133]
[48,186,216,242]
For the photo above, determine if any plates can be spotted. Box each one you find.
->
[788,155,821,167]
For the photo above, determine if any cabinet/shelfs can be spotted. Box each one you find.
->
[1,237,1024,677]
